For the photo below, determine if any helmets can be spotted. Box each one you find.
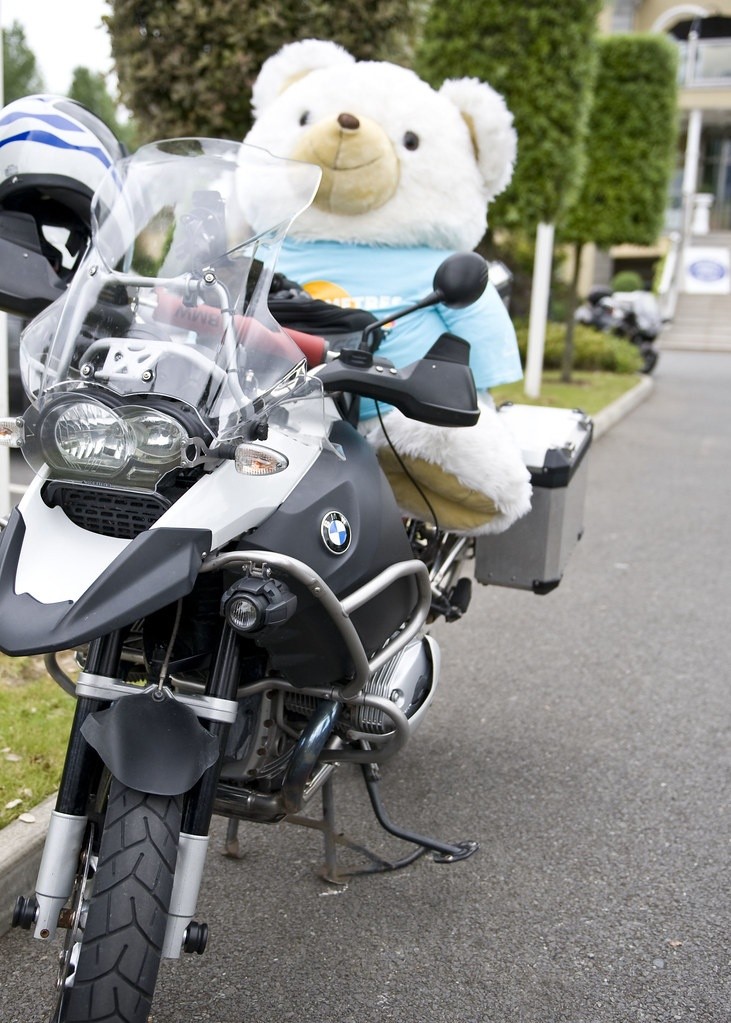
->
[0,94,134,272]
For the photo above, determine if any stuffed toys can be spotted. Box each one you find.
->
[233,40,534,538]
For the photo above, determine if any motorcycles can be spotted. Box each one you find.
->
[577,284,671,376]
[0,132,597,1023]
